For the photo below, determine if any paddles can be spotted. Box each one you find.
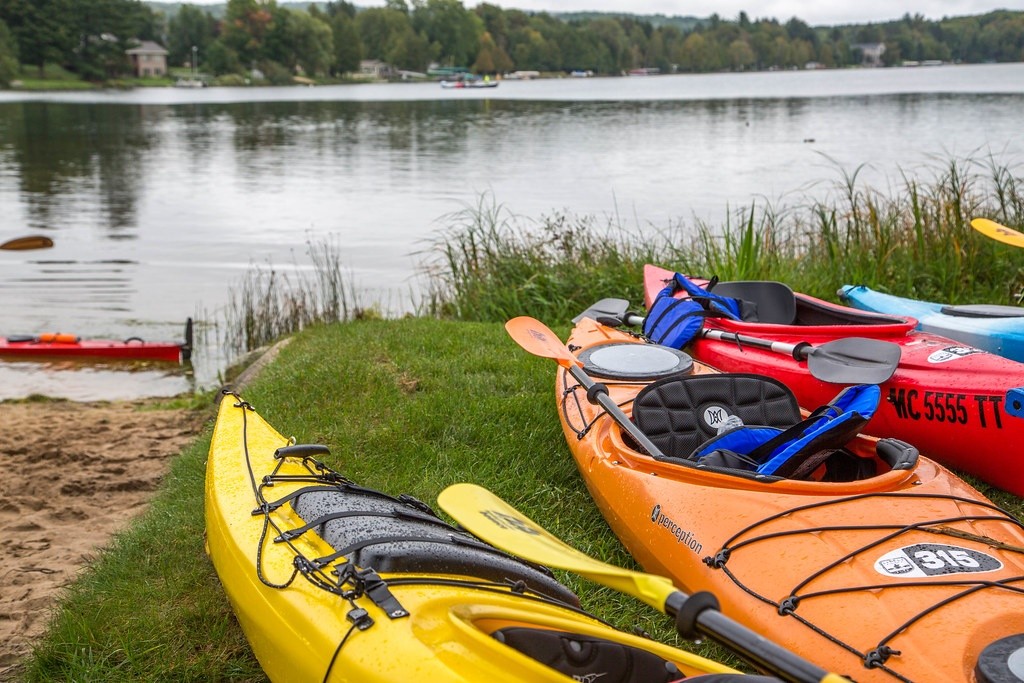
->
[970,218,1024,247]
[504,316,664,456]
[436,480,851,682]
[570,298,902,385]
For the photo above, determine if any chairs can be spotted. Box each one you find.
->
[630,373,804,462]
[710,281,796,325]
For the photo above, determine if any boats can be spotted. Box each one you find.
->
[0,332,186,366]
[440,79,500,89]
[201,385,784,683]
[552,316,1024,683]
[836,283,1024,363]
[638,260,1023,501]
